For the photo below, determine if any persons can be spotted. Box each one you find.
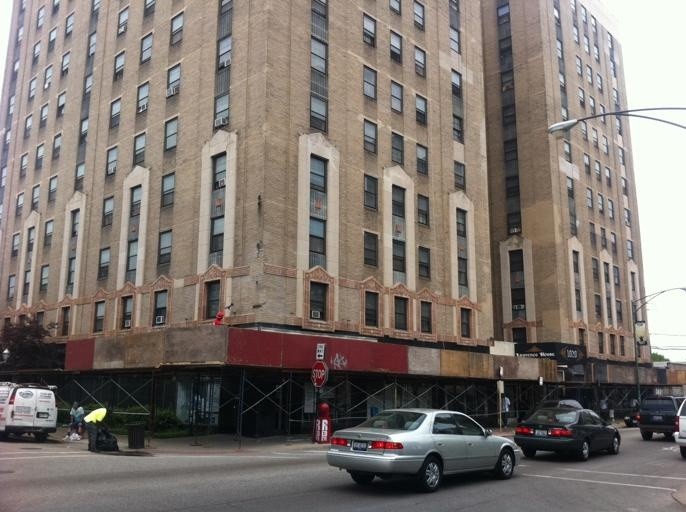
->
[63,401,84,440]
[630,397,639,408]
[501,394,510,429]
[600,396,607,411]
[608,396,615,422]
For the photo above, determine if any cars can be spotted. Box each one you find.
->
[623,408,639,427]
[514,407,621,461]
[326,408,520,492]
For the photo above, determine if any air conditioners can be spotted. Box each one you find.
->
[124,57,231,328]
[310,311,321,319]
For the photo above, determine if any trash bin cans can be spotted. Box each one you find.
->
[124,421,146,449]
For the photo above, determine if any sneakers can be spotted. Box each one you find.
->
[63,435,70,441]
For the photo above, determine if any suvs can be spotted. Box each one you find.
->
[636,395,686,458]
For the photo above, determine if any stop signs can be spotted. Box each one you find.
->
[310,360,329,389]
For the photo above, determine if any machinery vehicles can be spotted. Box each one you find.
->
[0,381,60,442]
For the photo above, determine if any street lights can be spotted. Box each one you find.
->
[545,107,685,137]
[631,287,686,401]
